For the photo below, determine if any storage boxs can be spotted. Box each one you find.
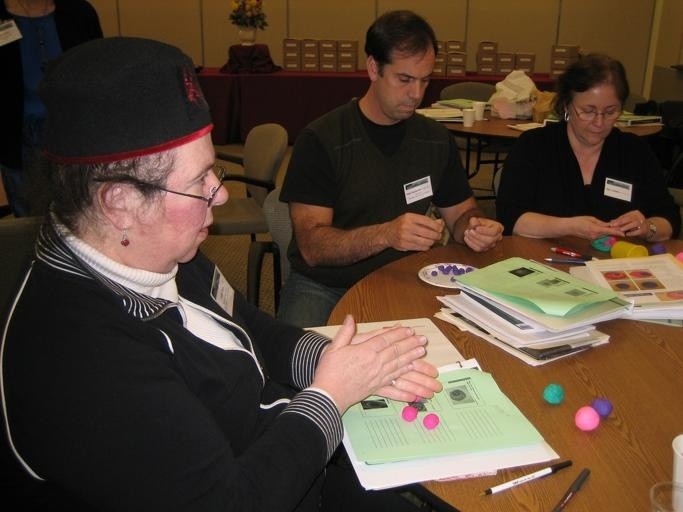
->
[279,36,359,74]
[431,40,582,78]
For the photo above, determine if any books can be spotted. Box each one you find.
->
[571,254,683,328]
[432,258,634,366]
[305,317,558,493]
[416,98,492,122]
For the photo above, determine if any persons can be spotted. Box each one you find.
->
[495,54,680,242]
[0,36,443,512]
[0,0,103,218]
[276,10,504,332]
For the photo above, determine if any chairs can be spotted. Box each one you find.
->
[439,79,508,188]
[0,213,49,328]
[243,183,293,323]
[200,121,288,243]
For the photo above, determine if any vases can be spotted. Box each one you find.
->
[237,23,257,48]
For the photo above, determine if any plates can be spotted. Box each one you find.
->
[418,262,478,289]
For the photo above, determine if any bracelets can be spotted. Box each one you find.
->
[646,220,656,241]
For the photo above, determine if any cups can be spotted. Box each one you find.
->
[471,102,484,121]
[462,108,475,126]
[648,479,682,512]
[671,434,682,511]
[610,241,648,258]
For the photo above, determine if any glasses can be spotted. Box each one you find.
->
[570,99,623,121]
[92,163,226,203]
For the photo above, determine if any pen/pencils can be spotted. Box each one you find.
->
[544,248,599,266]
[552,468,591,512]
[480,460,572,496]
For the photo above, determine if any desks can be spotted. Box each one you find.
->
[325,227,683,511]
[426,107,663,207]
[193,65,565,148]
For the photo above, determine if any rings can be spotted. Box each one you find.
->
[638,220,643,229]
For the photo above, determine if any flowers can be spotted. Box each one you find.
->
[228,0,271,30]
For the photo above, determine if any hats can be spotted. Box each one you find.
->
[39,36,216,165]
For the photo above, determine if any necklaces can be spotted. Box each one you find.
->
[17,0,48,71]
